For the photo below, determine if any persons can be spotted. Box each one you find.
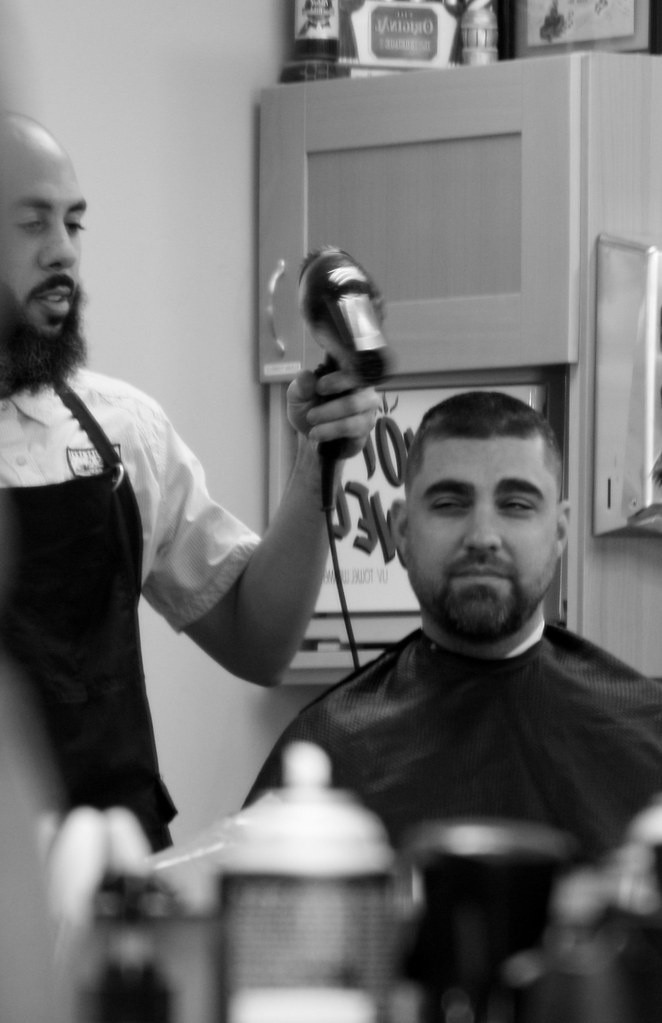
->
[0,109,382,1022]
[233,390,662,822]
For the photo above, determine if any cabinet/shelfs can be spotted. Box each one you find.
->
[255,51,661,688]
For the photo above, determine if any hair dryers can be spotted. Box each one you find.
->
[295,246,393,514]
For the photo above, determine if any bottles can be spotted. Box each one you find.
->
[295,0,339,60]
[461,0,498,67]
